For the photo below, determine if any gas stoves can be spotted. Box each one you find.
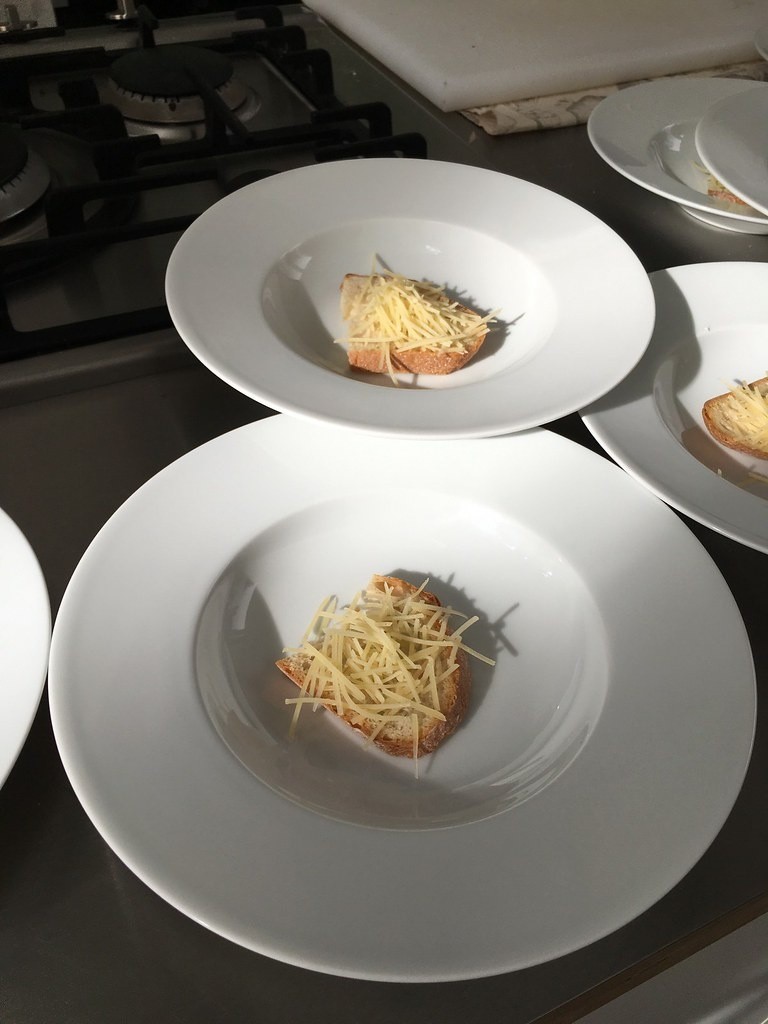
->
[0,0,448,403]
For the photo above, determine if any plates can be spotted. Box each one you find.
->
[694,85,768,218]
[162,156,660,439]
[49,416,760,983]
[586,76,767,234]
[576,260,767,554]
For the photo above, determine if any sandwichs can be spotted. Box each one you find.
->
[334,249,505,389]
[274,573,495,782]
[702,374,768,463]
